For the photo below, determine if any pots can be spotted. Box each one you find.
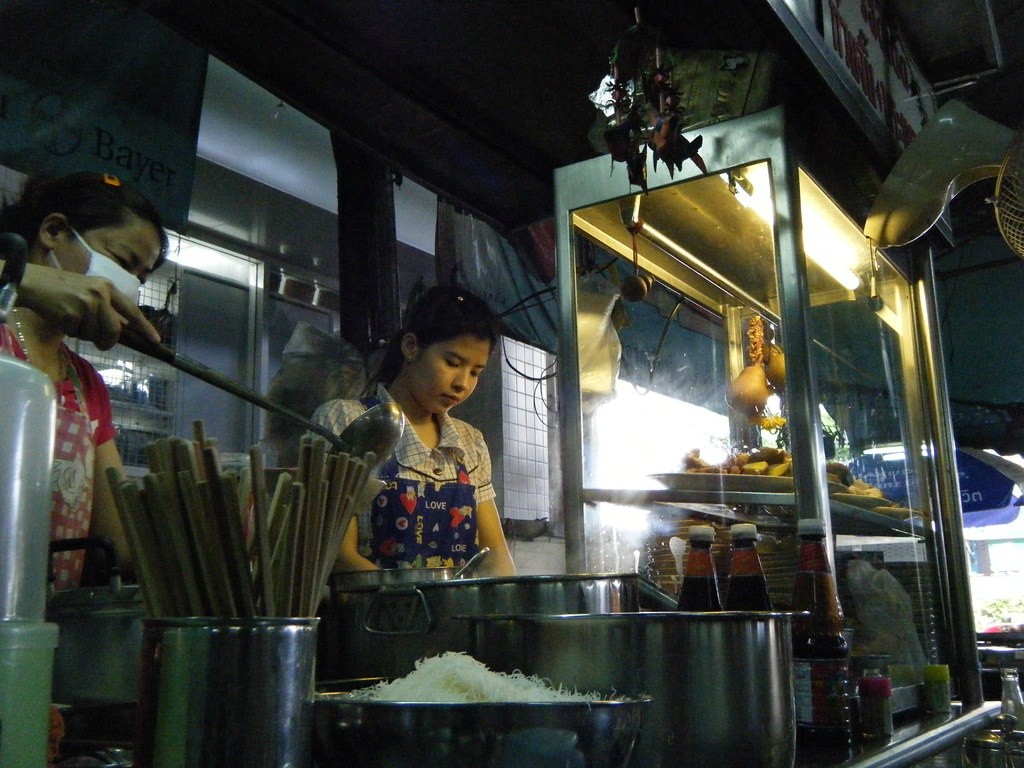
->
[320,574,678,679]
[450,610,811,768]
[46,537,148,707]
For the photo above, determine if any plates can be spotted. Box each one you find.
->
[872,506,923,521]
[647,472,849,495]
[645,519,799,609]
[835,551,937,665]
[831,493,891,509]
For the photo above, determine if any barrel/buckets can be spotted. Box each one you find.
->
[0,354,61,768]
[960,714,1024,768]
[141,617,321,768]
[327,568,467,584]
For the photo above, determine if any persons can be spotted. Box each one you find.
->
[0,170,168,595]
[268,323,366,467]
[305,287,516,578]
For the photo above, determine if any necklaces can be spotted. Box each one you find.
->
[12,307,29,363]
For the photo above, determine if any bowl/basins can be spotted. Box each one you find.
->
[314,691,656,768]
[263,468,388,512]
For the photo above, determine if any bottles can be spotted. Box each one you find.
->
[922,664,951,721]
[1000,668,1024,731]
[727,523,773,611]
[858,653,892,747]
[791,519,851,764]
[675,525,722,612]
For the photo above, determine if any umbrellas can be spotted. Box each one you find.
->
[846,445,1024,528]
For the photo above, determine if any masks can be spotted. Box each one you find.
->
[47,221,140,324]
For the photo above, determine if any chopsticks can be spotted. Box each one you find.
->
[105,420,378,617]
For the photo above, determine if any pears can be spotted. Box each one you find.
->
[725,363,768,413]
[766,353,785,387]
